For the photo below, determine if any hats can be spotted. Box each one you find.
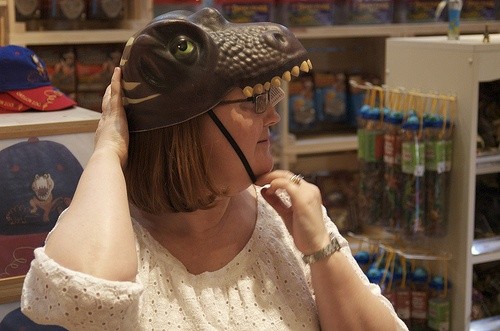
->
[0,43,76,114]
[115,7,314,134]
[0,138,85,235]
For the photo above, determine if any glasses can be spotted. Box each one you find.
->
[221,87,272,114]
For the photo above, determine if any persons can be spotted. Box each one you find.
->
[21,8,408,331]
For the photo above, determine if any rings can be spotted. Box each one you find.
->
[290,173,304,184]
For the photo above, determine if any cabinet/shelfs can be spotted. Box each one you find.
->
[0,0,500,331]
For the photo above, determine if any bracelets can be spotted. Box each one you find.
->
[302,232,341,265]
